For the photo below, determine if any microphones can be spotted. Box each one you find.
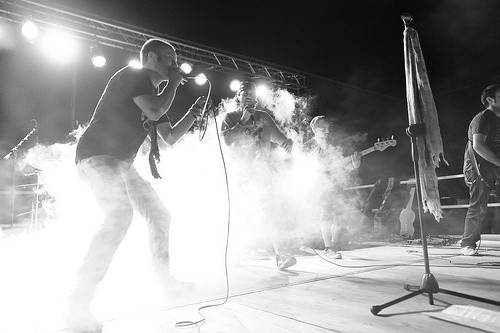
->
[250,115,254,121]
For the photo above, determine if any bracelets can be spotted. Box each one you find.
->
[237,122,244,127]
[188,103,203,119]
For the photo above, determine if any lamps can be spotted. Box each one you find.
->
[88,40,108,69]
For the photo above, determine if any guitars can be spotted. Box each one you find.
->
[329,136,397,170]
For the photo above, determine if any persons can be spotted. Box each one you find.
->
[221,81,297,269]
[302,116,363,259]
[459,85,500,256]
[62,39,212,332]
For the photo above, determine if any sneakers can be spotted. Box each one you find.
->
[460,246,478,256]
[276,253,297,271]
[324,248,342,259]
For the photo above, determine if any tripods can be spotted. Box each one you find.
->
[371,12,500,315]
